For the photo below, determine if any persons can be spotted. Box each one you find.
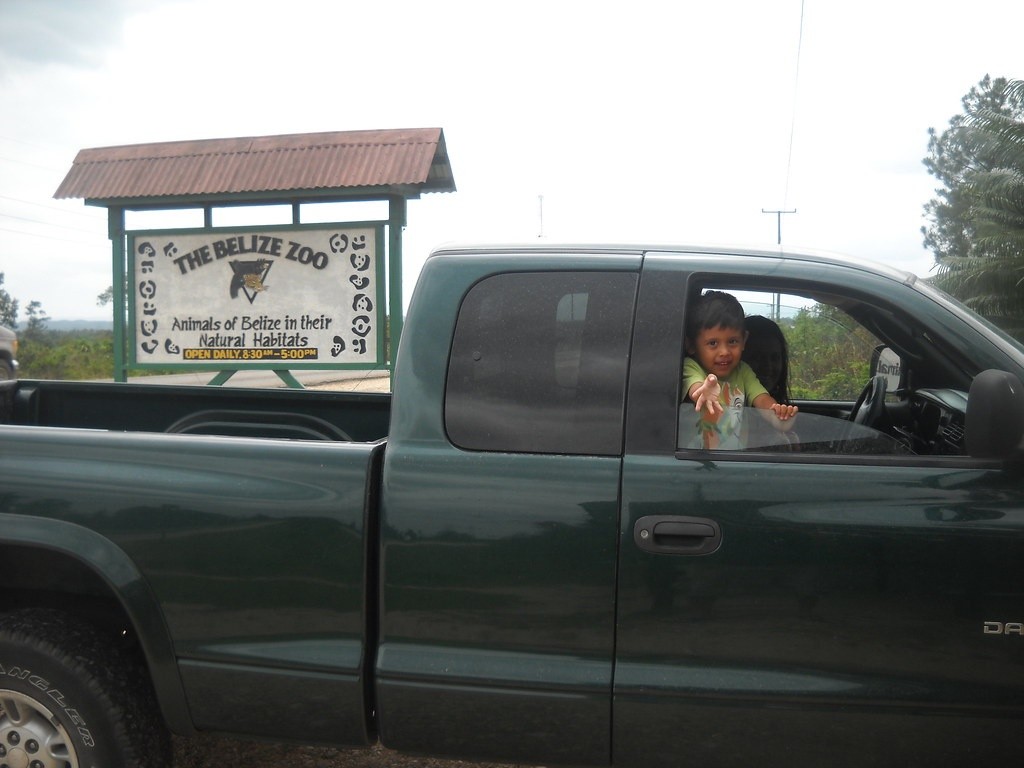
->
[681,291,798,452]
[742,314,801,452]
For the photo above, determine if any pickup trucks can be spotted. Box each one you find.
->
[0,238,1024,767]
